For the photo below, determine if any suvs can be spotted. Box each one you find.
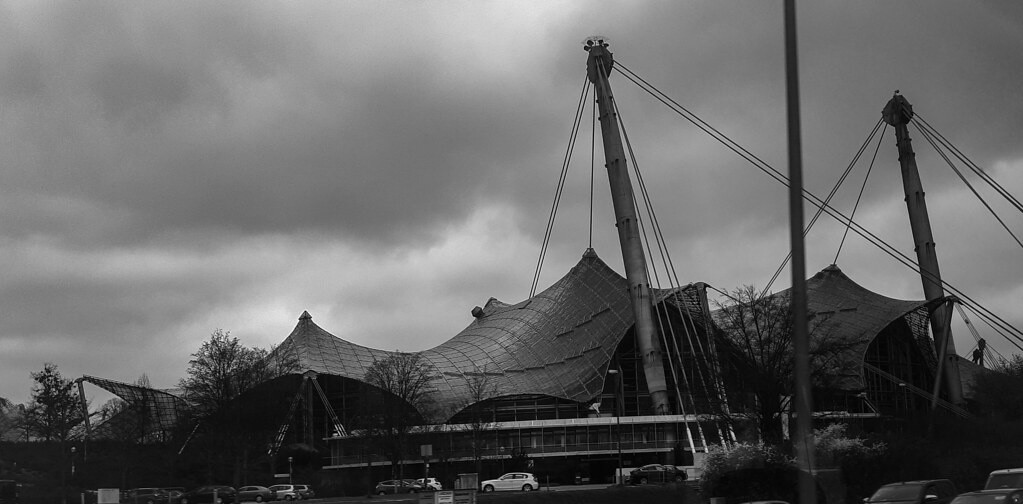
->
[296,484,315,500]
[481,472,539,492]
[177,486,237,504]
[268,484,298,502]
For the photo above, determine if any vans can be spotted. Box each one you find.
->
[984,468,1023,490]
[861,479,956,504]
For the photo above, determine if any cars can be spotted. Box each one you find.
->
[376,477,443,496]
[950,488,1023,504]
[624,464,688,485]
[119,487,184,504]
[237,485,275,503]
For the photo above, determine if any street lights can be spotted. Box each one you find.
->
[288,456,293,484]
[71,446,77,480]
[500,447,506,474]
